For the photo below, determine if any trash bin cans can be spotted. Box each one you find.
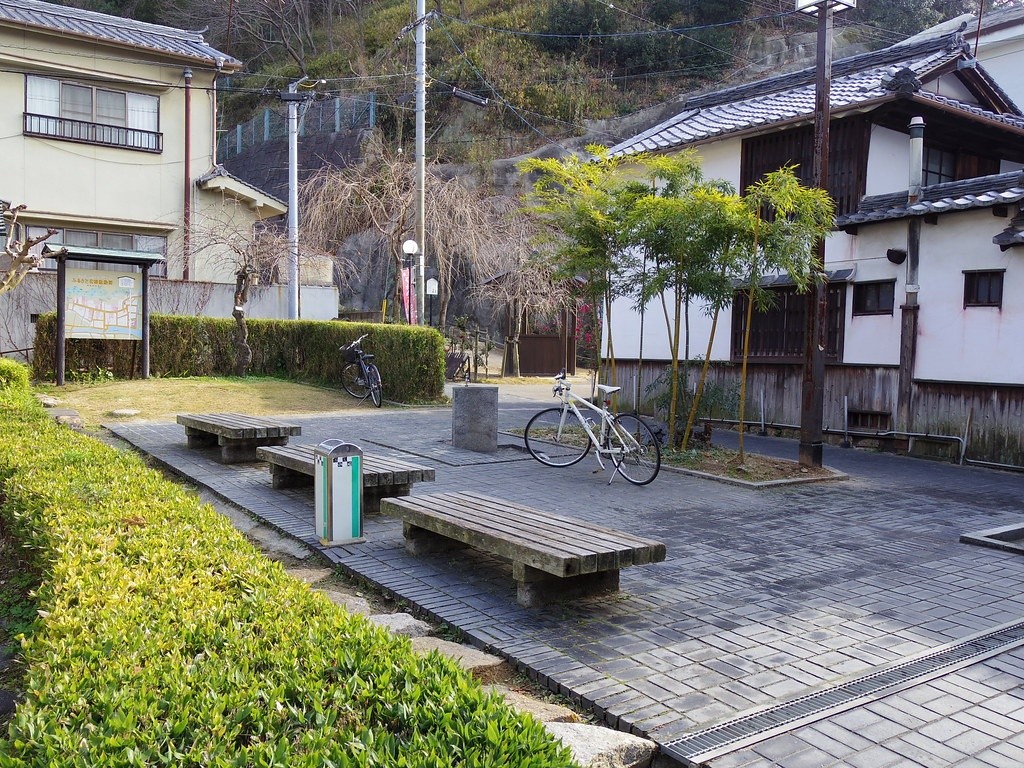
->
[314,438,366,547]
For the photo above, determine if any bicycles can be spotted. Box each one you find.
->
[524,367,660,486]
[339,334,382,408]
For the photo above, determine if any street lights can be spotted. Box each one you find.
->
[793,0,857,471]
[401,239,419,327]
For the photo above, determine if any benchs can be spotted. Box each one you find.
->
[176,414,302,463]
[258,445,435,515]
[381,491,668,605]
